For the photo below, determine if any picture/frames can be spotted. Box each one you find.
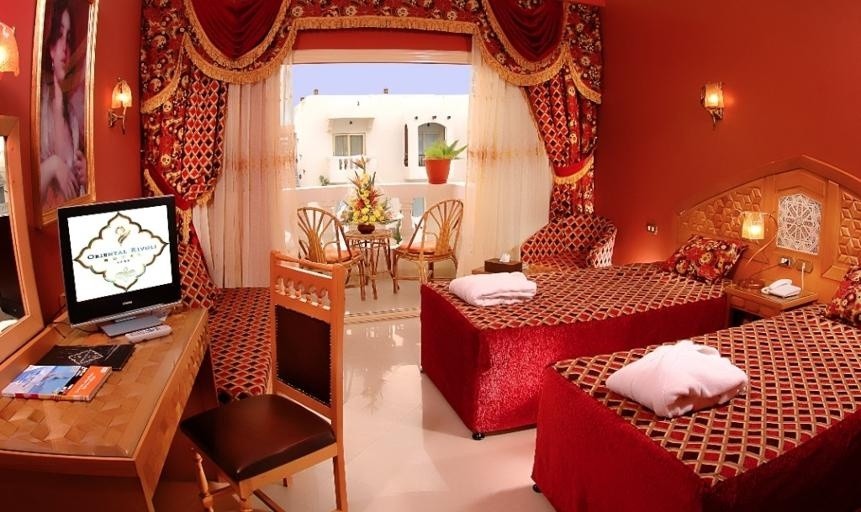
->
[31,0,99,233]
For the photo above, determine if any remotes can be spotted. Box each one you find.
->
[124,324,174,344]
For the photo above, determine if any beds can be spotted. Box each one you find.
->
[421,259,740,439]
[530,302,861,511]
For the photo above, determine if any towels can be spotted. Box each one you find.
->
[449,271,538,307]
[606,339,749,418]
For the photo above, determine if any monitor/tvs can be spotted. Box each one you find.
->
[56,194,184,338]
[0,215,25,320]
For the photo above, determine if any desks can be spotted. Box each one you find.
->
[345,230,400,299]
[0,307,227,511]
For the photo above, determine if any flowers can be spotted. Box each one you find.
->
[338,156,400,225]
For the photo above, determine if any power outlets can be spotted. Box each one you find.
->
[795,259,813,273]
[780,257,792,267]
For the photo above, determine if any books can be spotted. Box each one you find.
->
[37,343,134,371]
[1,364,113,402]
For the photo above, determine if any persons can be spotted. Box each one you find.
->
[41,2,88,208]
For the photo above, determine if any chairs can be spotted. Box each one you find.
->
[178,252,349,512]
[297,207,366,301]
[392,198,463,293]
[520,214,617,268]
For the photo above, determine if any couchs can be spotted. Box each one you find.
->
[180,241,271,408]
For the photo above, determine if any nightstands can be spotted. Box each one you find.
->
[724,279,818,330]
[472,259,557,275]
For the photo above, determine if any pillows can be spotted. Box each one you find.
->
[662,235,749,286]
[821,259,861,329]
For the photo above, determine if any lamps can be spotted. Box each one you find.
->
[737,212,788,289]
[108,75,132,132]
[700,80,725,125]
[0,20,20,76]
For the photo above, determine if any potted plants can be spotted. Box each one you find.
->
[422,139,468,184]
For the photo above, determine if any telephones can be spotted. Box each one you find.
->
[760,279,802,298]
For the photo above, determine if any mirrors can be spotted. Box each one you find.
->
[0,114,46,365]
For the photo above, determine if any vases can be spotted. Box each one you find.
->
[358,224,375,234]
